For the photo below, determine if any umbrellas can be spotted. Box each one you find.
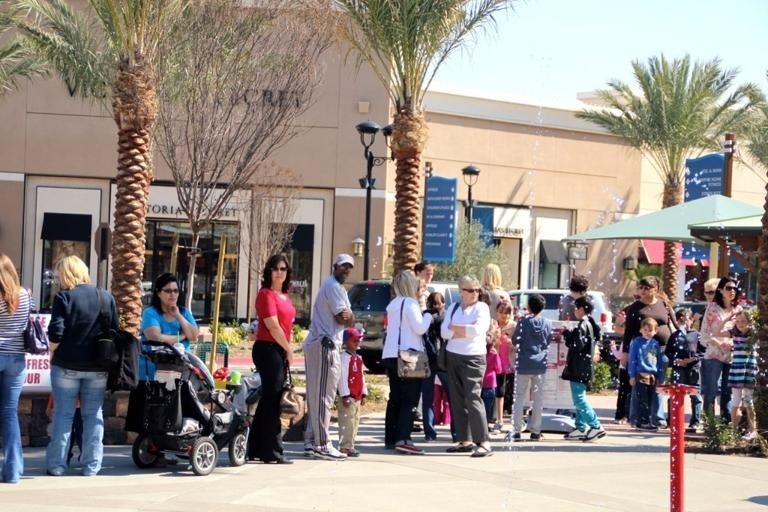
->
[560,193,763,246]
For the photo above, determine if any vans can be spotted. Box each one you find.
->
[671,301,706,317]
[506,286,616,337]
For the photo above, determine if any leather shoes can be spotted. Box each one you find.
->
[247,452,264,458]
[263,453,295,465]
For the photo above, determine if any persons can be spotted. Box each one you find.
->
[411,263,433,310]
[566,297,605,443]
[665,308,705,431]
[1,253,35,484]
[480,264,511,318]
[421,292,451,442]
[303,254,354,457]
[629,318,663,431]
[699,277,743,426]
[510,295,553,440]
[138,272,198,466]
[561,277,599,321]
[440,275,491,457]
[247,254,297,463]
[381,270,436,456]
[482,336,501,431]
[728,310,757,441]
[615,276,682,424]
[47,381,84,466]
[609,338,631,425]
[337,328,367,456]
[704,278,720,302]
[49,255,120,476]
[493,295,516,417]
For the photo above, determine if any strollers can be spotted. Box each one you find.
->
[124,339,253,475]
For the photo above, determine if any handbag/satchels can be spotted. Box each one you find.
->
[438,302,461,370]
[280,360,301,415]
[98,289,139,392]
[126,354,183,435]
[398,298,430,378]
[24,288,49,354]
[663,301,679,333]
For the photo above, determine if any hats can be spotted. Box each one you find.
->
[335,253,354,269]
[344,327,365,342]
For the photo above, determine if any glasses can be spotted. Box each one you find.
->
[273,266,289,272]
[723,285,739,292]
[462,288,482,293]
[162,288,180,293]
[706,290,716,296]
[639,285,659,291]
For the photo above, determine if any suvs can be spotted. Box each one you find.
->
[346,279,460,370]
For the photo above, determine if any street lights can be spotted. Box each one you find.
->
[456,165,481,229]
[355,119,395,281]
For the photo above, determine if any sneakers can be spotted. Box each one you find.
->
[686,423,701,432]
[510,434,522,441]
[395,440,425,454]
[341,446,361,458]
[314,447,349,461]
[565,431,589,440]
[635,425,658,433]
[303,447,316,457]
[742,431,759,440]
[580,427,606,442]
[530,432,542,440]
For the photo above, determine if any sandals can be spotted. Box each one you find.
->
[447,442,476,452]
[472,444,495,457]
